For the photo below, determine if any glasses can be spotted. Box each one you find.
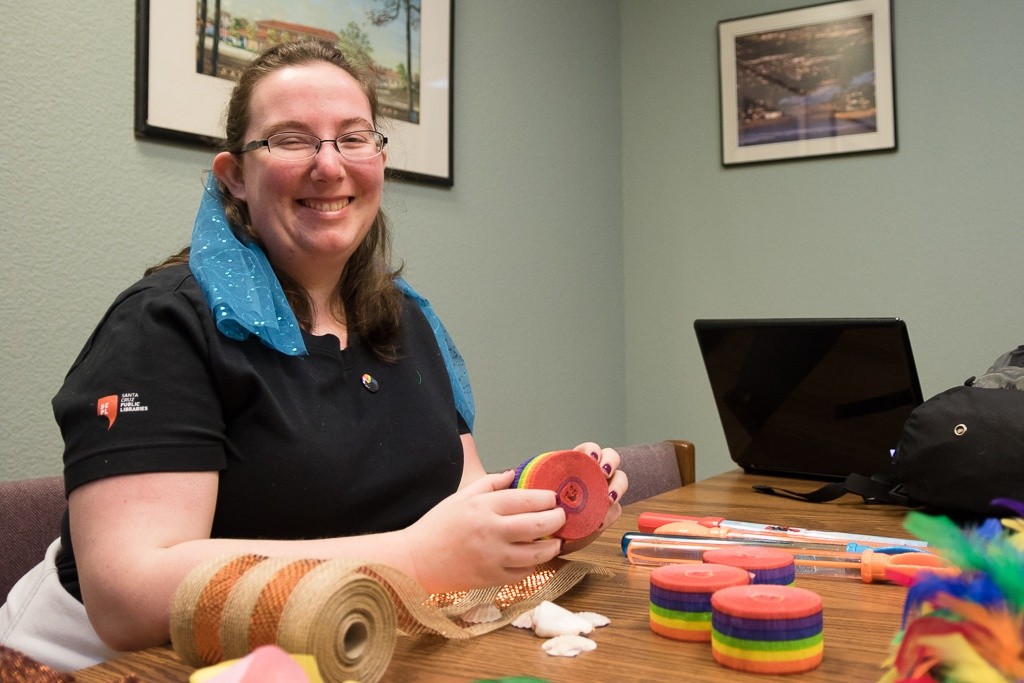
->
[237,129,389,162]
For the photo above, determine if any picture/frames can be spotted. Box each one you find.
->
[717,0,898,167]
[134,0,457,190]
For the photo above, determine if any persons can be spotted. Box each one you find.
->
[1,34,629,674]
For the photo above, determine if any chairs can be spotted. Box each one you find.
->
[0,477,67,608]
[486,441,696,525]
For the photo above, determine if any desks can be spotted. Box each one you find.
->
[67,467,1024,683]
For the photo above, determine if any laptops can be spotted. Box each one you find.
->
[692,316,927,483]
